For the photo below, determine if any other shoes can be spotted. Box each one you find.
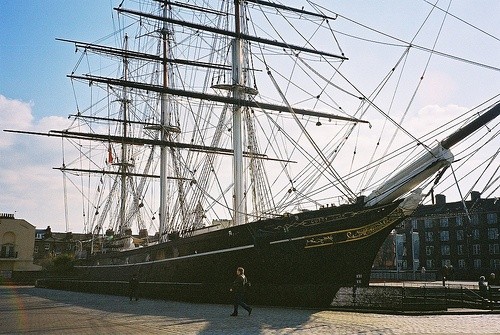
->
[231,312,238,316]
[249,307,252,315]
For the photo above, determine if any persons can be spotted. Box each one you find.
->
[129,274,139,302]
[230,267,253,317]
[479,275,487,308]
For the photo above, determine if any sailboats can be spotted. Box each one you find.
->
[38,0,500,310]
